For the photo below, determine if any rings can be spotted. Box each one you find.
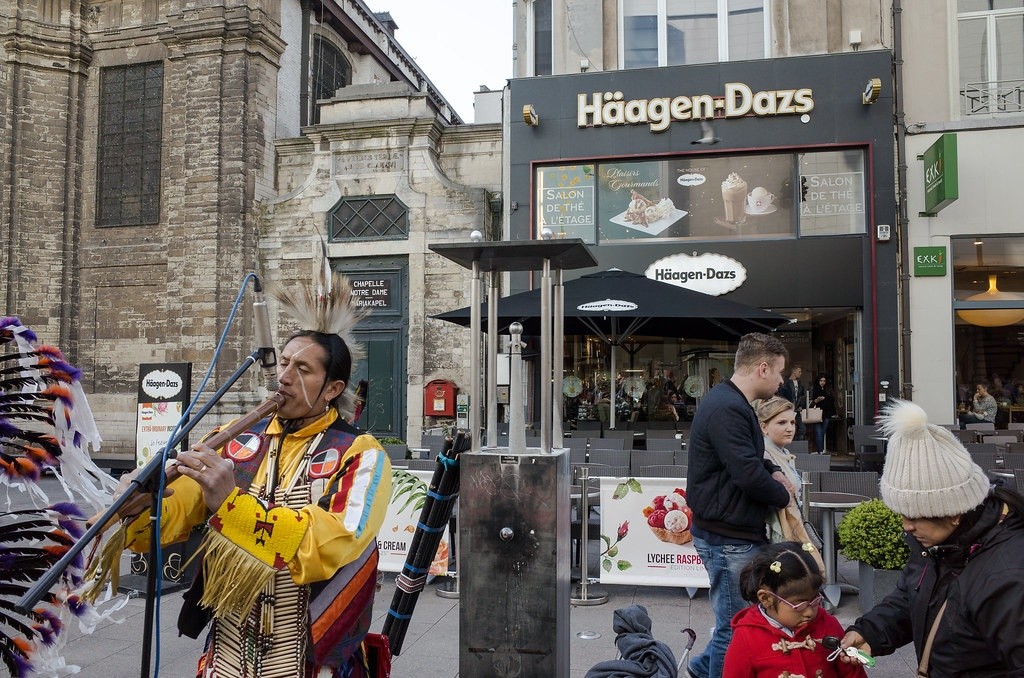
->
[115,494,120,499]
[201,466,206,473]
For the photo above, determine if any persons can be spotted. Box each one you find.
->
[810,375,839,455]
[838,393,1024,678]
[709,368,721,389]
[960,380,997,431]
[720,542,871,678]
[956,373,1024,430]
[686,333,796,678]
[113,329,392,678]
[781,363,805,440]
[752,395,825,581]
[578,370,684,421]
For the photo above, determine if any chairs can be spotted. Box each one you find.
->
[374,421,1024,581]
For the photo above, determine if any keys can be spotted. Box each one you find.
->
[814,637,869,664]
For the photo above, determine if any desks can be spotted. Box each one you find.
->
[570,462,612,485]
[986,469,1015,490]
[997,405,1024,429]
[794,490,873,614]
[868,433,889,453]
[956,409,970,419]
[409,444,430,459]
[975,428,1024,445]
[567,484,601,580]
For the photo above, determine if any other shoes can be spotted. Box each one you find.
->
[685,666,700,678]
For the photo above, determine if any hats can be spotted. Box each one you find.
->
[873,397,991,518]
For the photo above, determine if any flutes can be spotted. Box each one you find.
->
[86,391,286,537]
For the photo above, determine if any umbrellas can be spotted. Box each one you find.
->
[429,267,797,430]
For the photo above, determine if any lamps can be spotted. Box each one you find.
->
[523,104,539,127]
[863,78,881,105]
[958,274,1024,326]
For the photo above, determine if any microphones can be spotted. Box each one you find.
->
[253,277,279,391]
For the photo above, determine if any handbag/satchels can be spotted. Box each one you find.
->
[804,521,825,552]
[800,400,822,423]
[973,410,988,420]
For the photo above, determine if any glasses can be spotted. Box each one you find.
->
[766,590,824,613]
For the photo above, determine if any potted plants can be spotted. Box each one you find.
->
[838,500,908,614]
[378,436,412,459]
